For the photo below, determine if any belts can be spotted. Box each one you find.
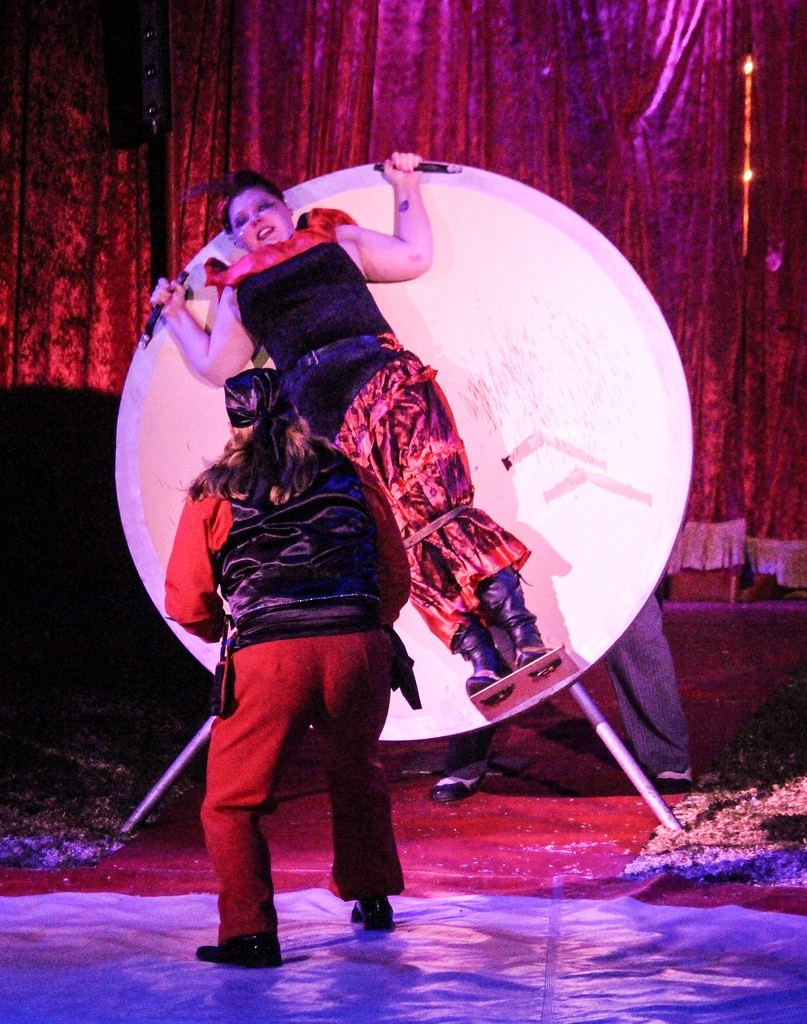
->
[282,336,383,389]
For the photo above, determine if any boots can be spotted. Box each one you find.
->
[453,620,515,704]
[475,570,560,677]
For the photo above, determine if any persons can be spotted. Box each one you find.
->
[432,593,692,802]
[164,367,422,969]
[149,150,559,705]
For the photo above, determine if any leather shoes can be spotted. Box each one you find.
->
[350,892,395,930]
[194,935,280,968]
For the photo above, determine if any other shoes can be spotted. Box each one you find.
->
[430,770,483,803]
[655,764,690,795]
